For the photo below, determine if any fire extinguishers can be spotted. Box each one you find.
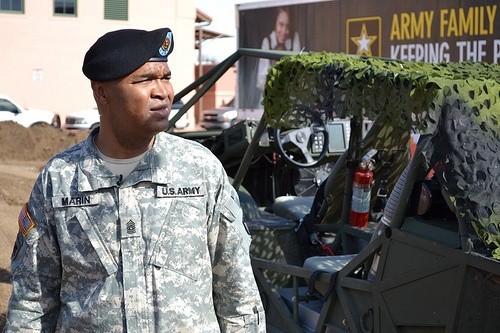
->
[348,149,377,229]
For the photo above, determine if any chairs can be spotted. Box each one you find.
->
[272,111,411,230]
[303,122,414,303]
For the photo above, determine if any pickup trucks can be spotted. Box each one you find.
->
[198,94,238,131]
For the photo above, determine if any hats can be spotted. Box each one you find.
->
[82,28,174,83]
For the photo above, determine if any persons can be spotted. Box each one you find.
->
[4,27,266,333]
[256,8,300,109]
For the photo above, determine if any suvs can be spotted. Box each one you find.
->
[65,101,190,131]
[0,94,59,128]
[161,43,496,332]
